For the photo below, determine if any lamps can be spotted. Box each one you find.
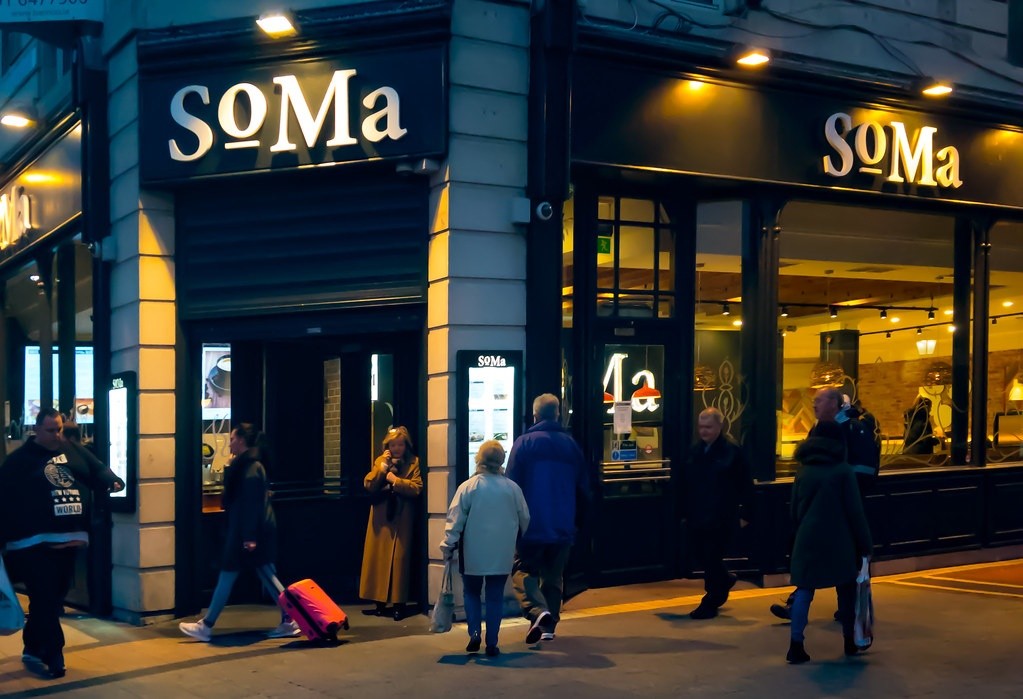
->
[887,332,891,338]
[781,307,789,317]
[0,104,39,129]
[992,318,997,324]
[631,345,662,399]
[727,41,773,68]
[602,391,616,403]
[722,305,730,316]
[830,308,838,318]
[929,311,934,319]
[255,5,301,41]
[880,309,888,319]
[911,75,954,98]
[917,328,922,335]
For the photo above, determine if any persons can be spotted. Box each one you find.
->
[358,427,424,621]
[178,424,301,642]
[503,393,593,644]
[0,408,125,681]
[902,397,940,454]
[439,441,530,656]
[687,405,757,620]
[770,374,881,664]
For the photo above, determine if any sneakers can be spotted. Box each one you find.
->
[526,609,551,644]
[540,628,554,639]
[268,620,301,637]
[179,619,211,642]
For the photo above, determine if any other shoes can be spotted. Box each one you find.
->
[690,604,718,618]
[716,573,736,606]
[845,639,858,655]
[771,604,791,618]
[485,647,499,656]
[47,649,65,677]
[376,603,384,615]
[394,605,402,620]
[466,632,481,652]
[786,648,810,663]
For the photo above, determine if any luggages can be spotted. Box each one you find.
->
[244,541,349,646]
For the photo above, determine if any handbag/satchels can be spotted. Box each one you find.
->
[0,555,26,636]
[428,561,453,633]
[854,557,874,648]
